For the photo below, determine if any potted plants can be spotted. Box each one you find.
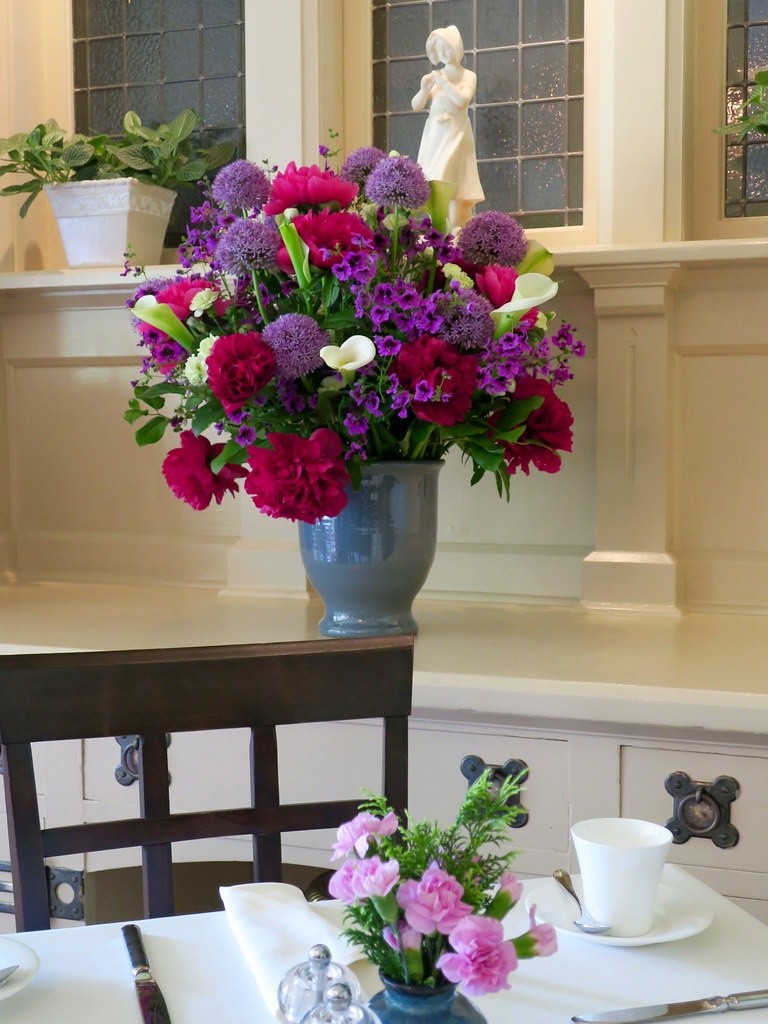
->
[0,109,238,267]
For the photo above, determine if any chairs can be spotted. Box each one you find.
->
[0,636,413,933]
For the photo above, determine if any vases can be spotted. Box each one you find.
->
[361,967,488,1024]
[298,459,445,637]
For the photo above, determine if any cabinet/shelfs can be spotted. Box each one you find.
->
[0,716,768,925]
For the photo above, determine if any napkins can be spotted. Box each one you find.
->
[220,883,357,1024]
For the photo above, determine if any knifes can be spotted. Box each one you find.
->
[570,989,768,1023]
[121,925,171,1024]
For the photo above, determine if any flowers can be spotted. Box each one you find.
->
[329,767,558,997]
[122,127,587,524]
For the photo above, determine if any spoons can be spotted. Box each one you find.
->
[550,869,611,933]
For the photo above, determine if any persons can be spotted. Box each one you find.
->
[410,24,484,233]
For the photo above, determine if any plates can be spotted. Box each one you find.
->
[0,936,40,1001]
[525,874,713,947]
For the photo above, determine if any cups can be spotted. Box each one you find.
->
[571,818,673,937]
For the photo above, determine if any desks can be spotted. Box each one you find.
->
[0,862,768,1024]
